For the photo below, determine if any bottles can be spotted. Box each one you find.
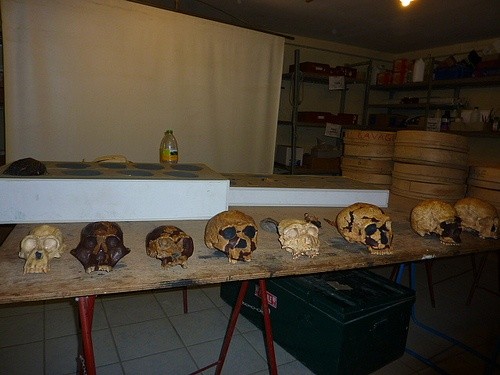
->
[160,130,178,167]
[440,111,451,131]
[411,58,425,83]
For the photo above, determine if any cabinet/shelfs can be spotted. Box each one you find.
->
[273,49,373,175]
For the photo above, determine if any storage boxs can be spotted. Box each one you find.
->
[478,54,500,68]
[221,268,417,374]
[369,114,390,128]
[276,145,303,166]
[297,111,358,125]
[288,62,357,79]
[376,58,409,84]
[303,151,335,169]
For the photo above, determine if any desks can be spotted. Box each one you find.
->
[0,194,500,375]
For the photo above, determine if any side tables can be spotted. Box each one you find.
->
[367,74,500,139]
